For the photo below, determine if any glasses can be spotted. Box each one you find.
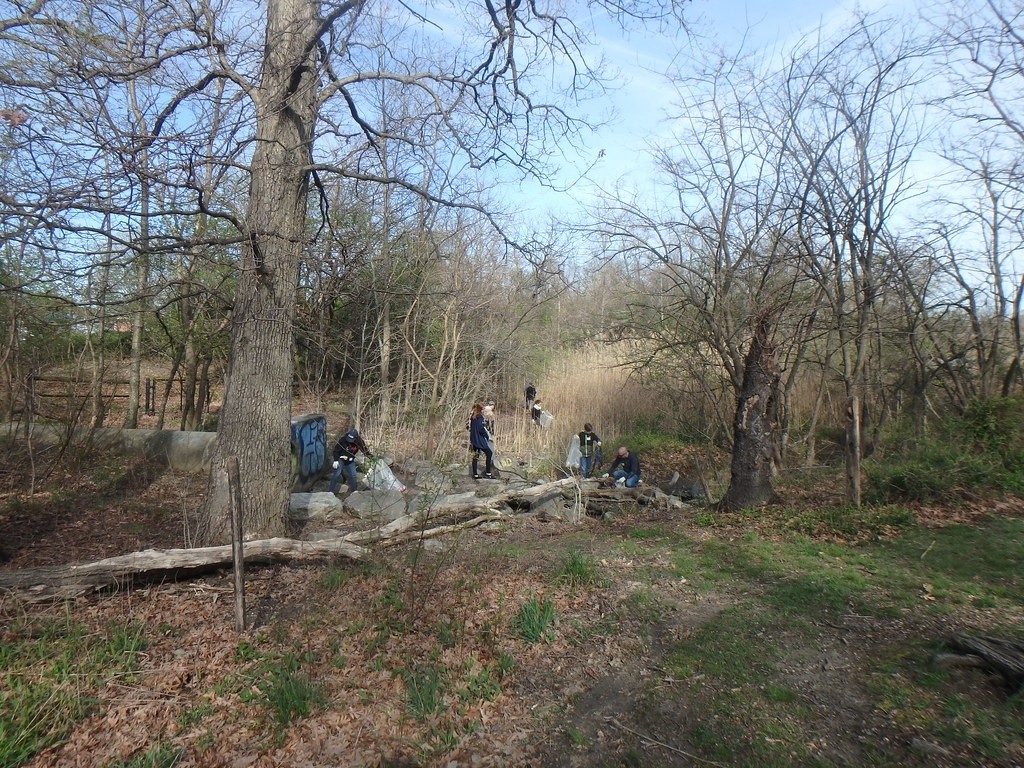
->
[619,454,624,457]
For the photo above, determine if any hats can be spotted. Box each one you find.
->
[346,429,358,442]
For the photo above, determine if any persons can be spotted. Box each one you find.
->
[466,383,544,482]
[332,429,375,497]
[577,423,603,478]
[601,448,641,487]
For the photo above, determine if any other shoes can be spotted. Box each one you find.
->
[473,475,486,478]
[486,475,496,479]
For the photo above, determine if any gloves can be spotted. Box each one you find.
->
[532,419,535,423]
[597,441,601,446]
[333,460,340,469]
[602,473,610,478]
[486,436,489,440]
[619,477,626,484]
